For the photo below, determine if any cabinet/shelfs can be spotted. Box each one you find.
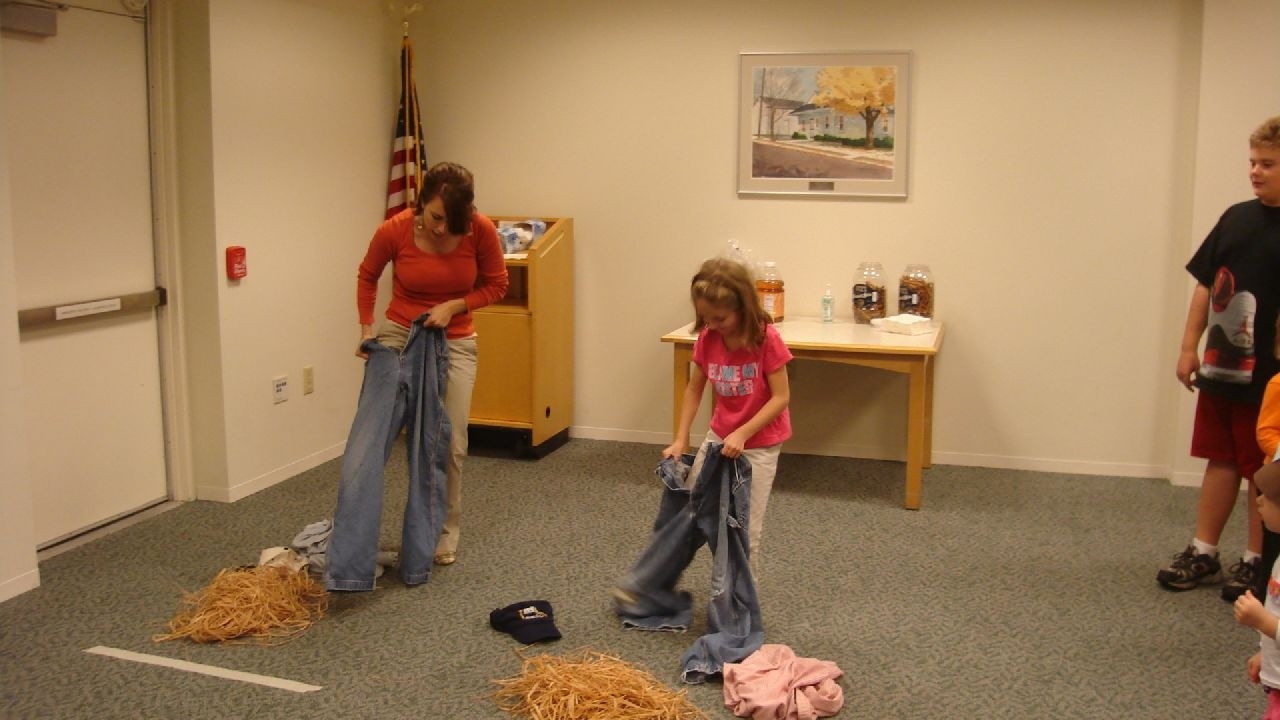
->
[468,216,575,459]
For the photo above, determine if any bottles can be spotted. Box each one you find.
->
[898,265,935,319]
[754,262,784,324]
[852,261,888,324]
[823,284,834,323]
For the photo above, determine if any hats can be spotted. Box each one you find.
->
[490,600,560,645]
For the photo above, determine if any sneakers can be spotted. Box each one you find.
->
[1221,556,1263,601]
[1157,545,1224,588]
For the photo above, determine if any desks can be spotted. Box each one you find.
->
[660,316,945,510]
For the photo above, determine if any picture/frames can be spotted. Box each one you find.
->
[736,50,912,197]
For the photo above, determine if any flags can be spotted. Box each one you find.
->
[383,18,430,221]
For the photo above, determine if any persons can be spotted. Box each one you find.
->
[1233,458,1280,720]
[1151,116,1280,601]
[1246,371,1280,598]
[326,161,509,595]
[609,256,802,681]
